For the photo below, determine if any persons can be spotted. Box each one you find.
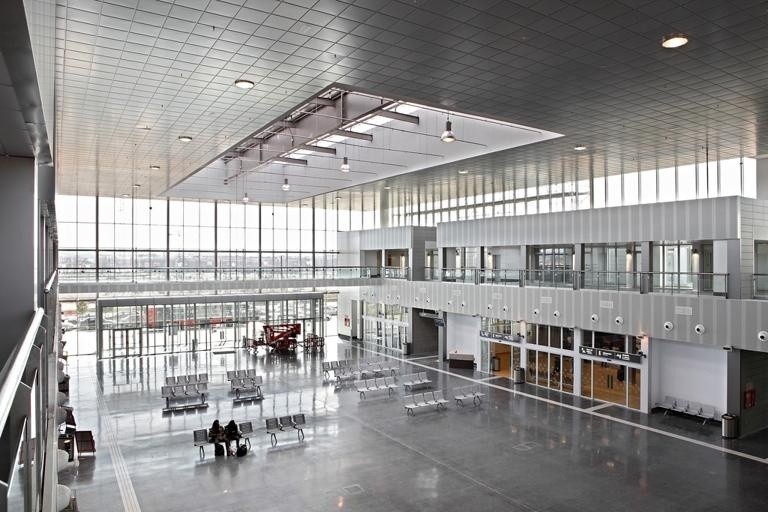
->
[223,420,241,457]
[210,420,231,457]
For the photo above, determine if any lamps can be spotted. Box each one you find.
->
[441,112,455,142]
[242,174,249,203]
[282,165,290,191]
[340,136,350,173]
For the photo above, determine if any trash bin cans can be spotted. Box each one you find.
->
[58,434,73,461]
[403,343,412,355]
[514,368,525,384]
[722,413,738,439]
[493,357,501,371]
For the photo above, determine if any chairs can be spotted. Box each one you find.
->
[655,396,716,428]
[322,356,486,417]
[161,370,307,462]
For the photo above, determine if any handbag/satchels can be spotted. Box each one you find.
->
[217,445,224,456]
[239,444,247,456]
